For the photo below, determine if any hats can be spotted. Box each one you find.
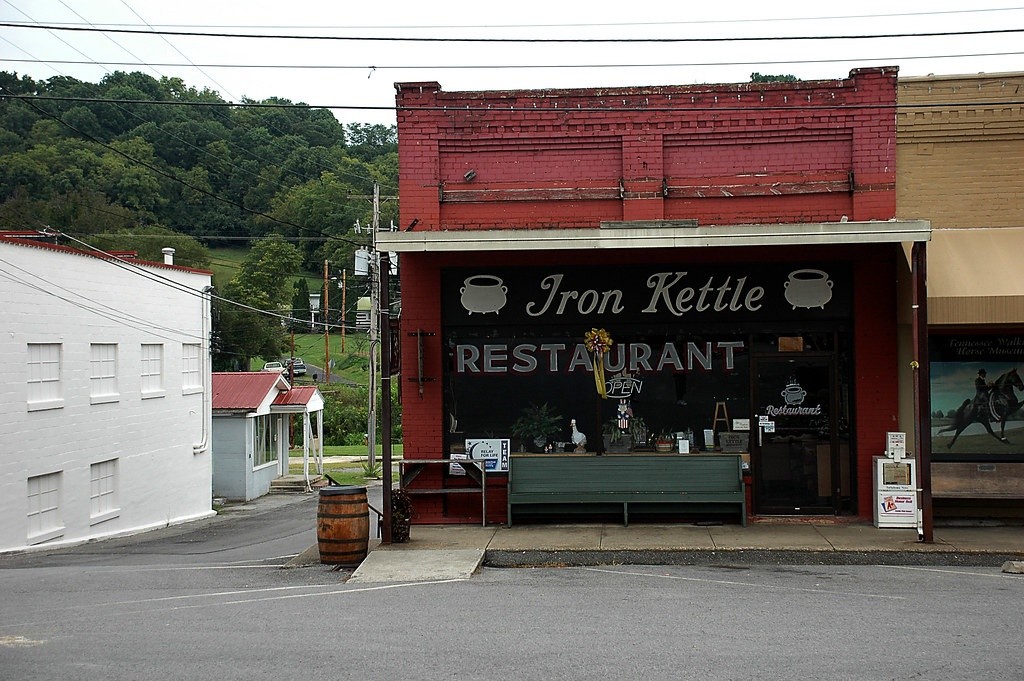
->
[977,368,986,374]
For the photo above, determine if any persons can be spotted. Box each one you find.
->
[975,368,995,418]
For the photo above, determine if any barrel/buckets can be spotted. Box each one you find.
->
[317,486,369,565]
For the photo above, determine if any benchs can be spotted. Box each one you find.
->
[507,453,748,529]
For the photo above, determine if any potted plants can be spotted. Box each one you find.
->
[392,488,415,540]
[602,417,644,454]
[652,425,676,452]
[511,402,563,453]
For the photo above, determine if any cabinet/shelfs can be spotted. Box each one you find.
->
[399,459,486,525]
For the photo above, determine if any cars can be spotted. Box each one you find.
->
[284,357,307,374]
[260,361,290,376]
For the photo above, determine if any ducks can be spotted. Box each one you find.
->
[569,418,586,450]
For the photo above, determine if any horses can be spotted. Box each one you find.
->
[936,365,1024,450]
